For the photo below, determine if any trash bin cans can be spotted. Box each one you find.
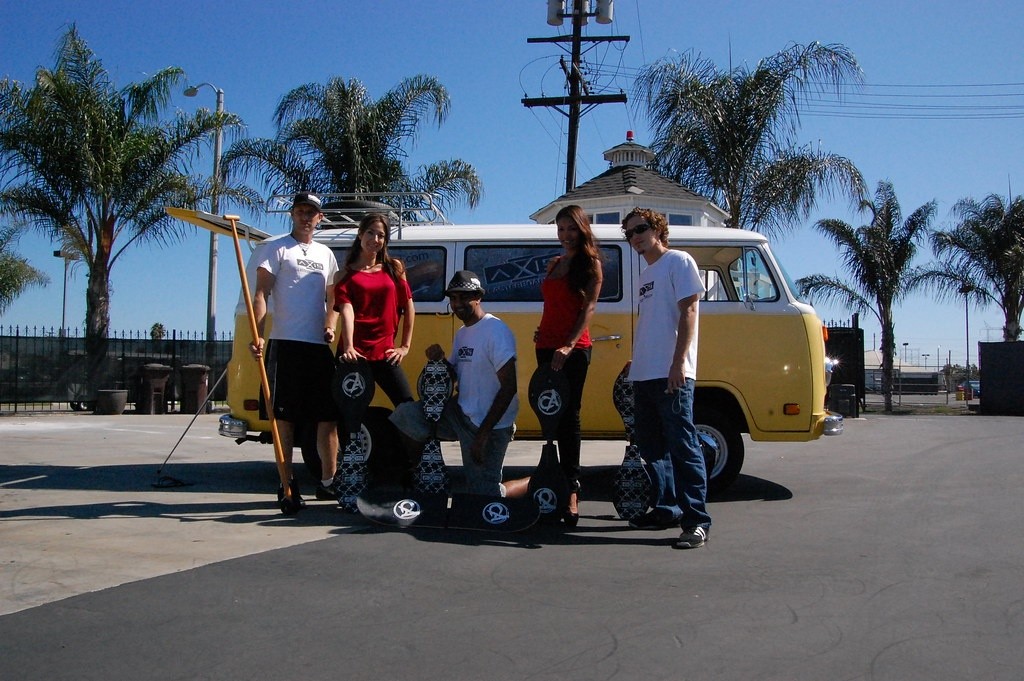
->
[133,363,173,414]
[180,363,211,414]
[828,383,857,419]
[955,386,965,401]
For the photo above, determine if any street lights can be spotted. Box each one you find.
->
[922,354,930,370]
[183,82,225,414]
[903,343,908,362]
[52,251,80,336]
[957,284,976,405]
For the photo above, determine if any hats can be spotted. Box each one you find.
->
[445,271,486,296]
[294,192,321,212]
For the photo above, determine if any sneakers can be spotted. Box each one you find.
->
[676,526,710,548]
[629,507,682,530]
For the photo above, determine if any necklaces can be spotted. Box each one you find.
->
[289,232,311,256]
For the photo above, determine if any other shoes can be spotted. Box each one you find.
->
[278,477,304,516]
[564,506,579,529]
[316,478,338,501]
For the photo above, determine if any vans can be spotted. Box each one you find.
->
[159,198,843,502]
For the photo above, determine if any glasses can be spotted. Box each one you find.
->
[625,223,652,239]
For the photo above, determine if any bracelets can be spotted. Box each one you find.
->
[324,327,336,334]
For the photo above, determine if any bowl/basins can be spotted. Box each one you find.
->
[97,389,128,415]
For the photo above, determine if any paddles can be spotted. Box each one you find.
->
[221,213,295,502]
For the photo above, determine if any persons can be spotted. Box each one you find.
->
[249,193,343,511]
[333,214,416,499]
[621,207,712,548]
[385,271,584,500]
[532,205,603,528]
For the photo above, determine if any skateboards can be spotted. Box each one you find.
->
[329,358,374,516]
[609,366,652,521]
[357,490,542,535]
[528,361,569,526]
[411,360,454,496]
